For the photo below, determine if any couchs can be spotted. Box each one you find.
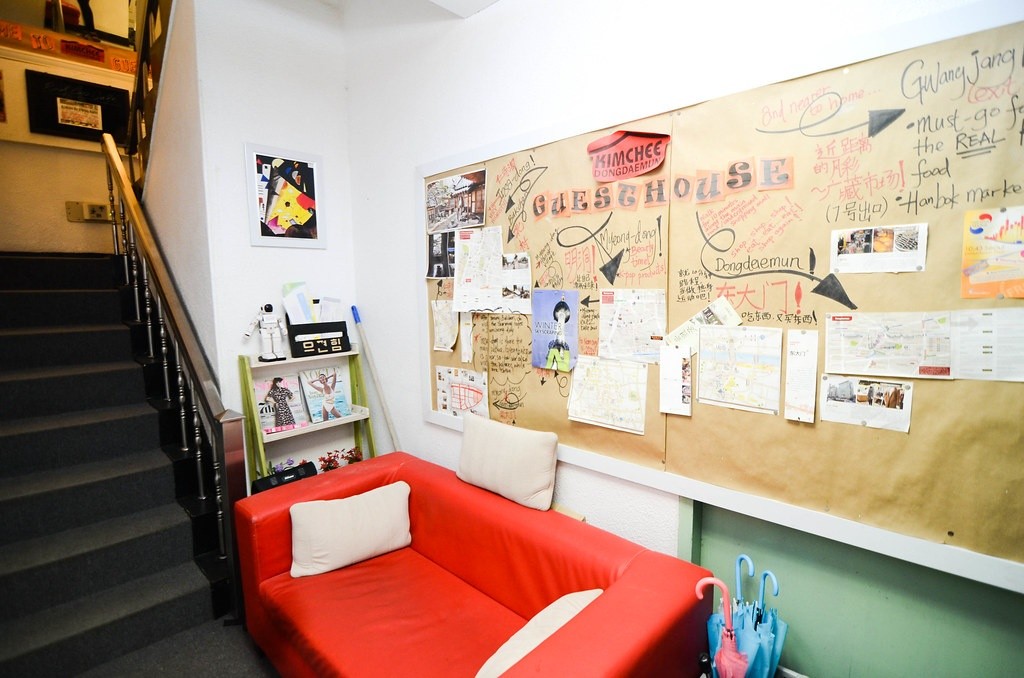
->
[235,452,713,678]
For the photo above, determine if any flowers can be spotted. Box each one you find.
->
[299,458,307,464]
[341,447,362,465]
[265,458,295,472]
[317,448,339,472]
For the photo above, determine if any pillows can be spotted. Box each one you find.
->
[291,480,411,577]
[454,411,558,512]
[476,588,602,678]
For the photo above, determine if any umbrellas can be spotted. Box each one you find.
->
[696,552,788,678]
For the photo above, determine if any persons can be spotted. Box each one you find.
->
[266,378,296,427]
[243,303,287,359]
[308,372,341,421]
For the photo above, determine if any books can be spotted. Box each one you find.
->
[252,374,308,433]
[299,366,351,424]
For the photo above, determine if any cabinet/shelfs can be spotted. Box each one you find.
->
[236,344,376,482]
